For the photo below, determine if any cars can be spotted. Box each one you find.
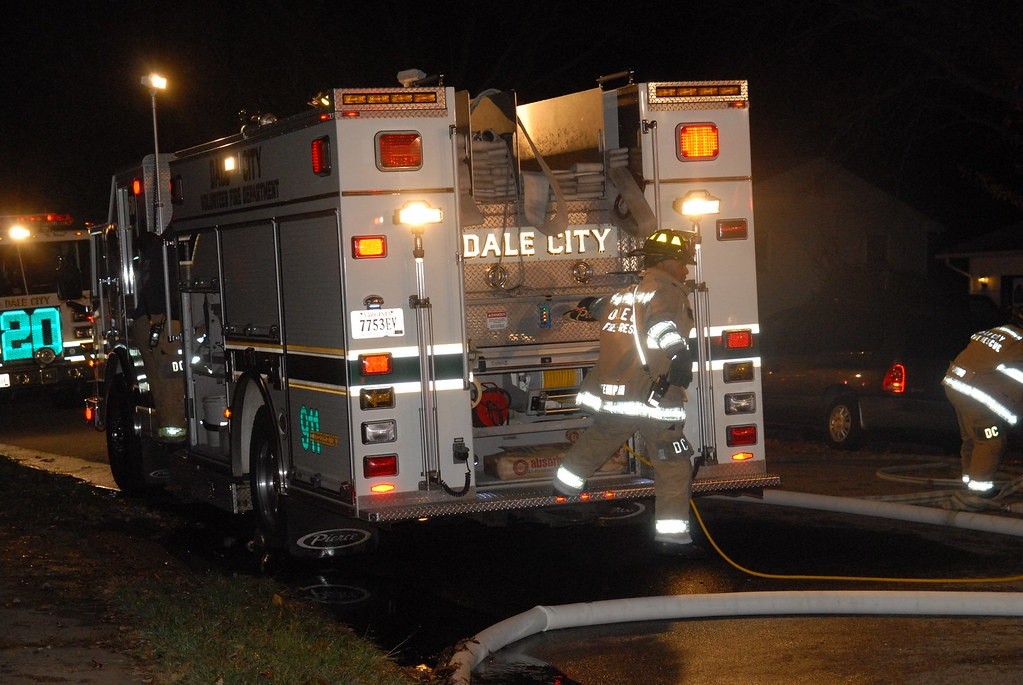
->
[753,294,969,447]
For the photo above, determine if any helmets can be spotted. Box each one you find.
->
[626,229,698,266]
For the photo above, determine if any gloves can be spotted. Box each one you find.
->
[570,297,599,323]
[668,349,692,389]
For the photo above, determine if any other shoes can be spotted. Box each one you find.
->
[552,487,570,499]
[968,486,1002,499]
[660,543,705,560]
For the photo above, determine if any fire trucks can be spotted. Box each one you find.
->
[95,65,784,586]
[0,212,111,428]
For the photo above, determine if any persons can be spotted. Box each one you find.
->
[940,316,1023,496]
[550,230,708,558]
